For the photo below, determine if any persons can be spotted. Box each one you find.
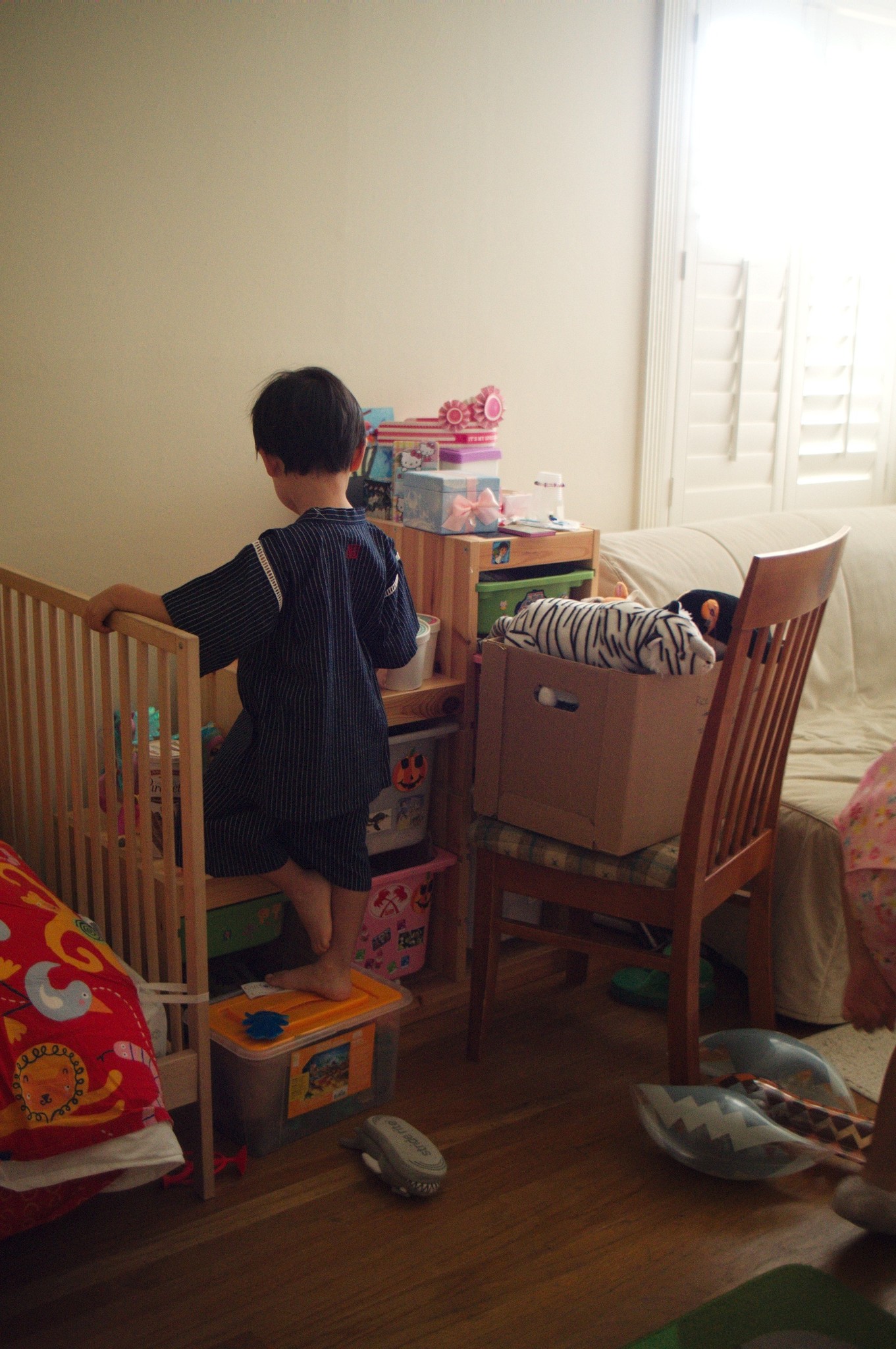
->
[81,367,420,1001]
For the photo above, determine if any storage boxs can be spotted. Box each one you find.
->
[403,471,500,535]
[180,568,783,1157]
[439,447,501,472]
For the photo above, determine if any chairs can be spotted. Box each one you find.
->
[465,528,853,1084]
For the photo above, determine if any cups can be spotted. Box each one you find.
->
[378,612,441,691]
[526,471,564,523]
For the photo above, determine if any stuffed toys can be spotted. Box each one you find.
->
[474,580,785,676]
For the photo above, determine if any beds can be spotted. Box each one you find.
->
[0,564,214,1246]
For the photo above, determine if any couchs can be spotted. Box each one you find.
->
[600,508,896,1025]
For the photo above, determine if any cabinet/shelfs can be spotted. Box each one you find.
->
[51,515,603,1053]
